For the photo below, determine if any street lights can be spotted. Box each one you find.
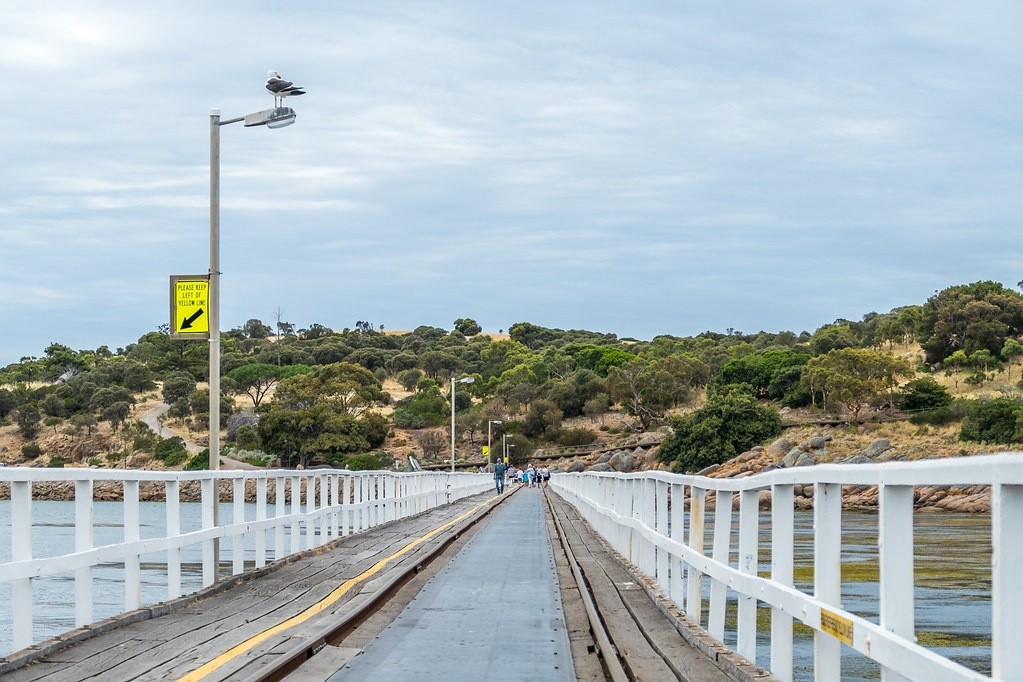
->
[450,377,474,472]
[503,434,513,465]
[488,419,503,473]
[208,68,310,582]
[507,443,516,467]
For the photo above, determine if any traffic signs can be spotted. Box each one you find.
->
[169,274,210,340]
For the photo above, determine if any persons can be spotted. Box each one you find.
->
[504,463,551,488]
[494,458,505,494]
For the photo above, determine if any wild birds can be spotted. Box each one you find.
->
[265,70,306,108]
